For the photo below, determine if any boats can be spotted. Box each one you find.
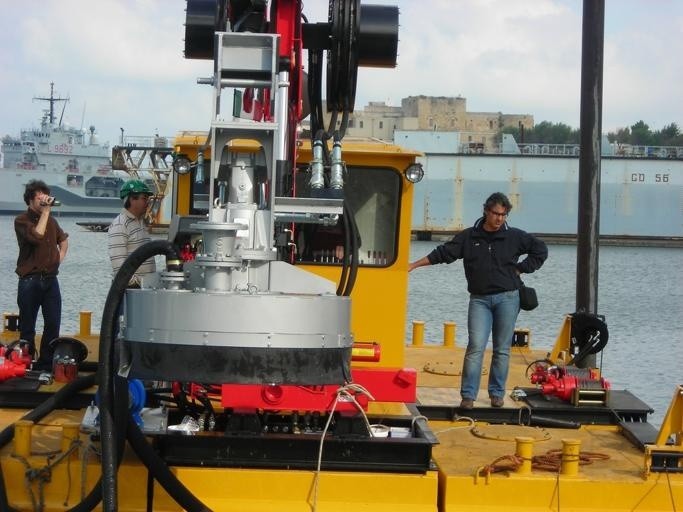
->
[50,144,177,233]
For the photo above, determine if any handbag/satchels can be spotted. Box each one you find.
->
[519,287,537,310]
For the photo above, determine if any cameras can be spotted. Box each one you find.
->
[47,195,61,207]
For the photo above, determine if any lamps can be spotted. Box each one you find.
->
[404,163,424,183]
[173,156,191,174]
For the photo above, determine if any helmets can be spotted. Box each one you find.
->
[120,180,153,198]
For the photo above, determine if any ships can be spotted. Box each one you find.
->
[0,82,112,212]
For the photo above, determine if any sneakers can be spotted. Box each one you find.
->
[490,396,504,407]
[461,398,473,410]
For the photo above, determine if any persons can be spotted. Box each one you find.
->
[14,181,68,363]
[290,187,359,259]
[408,191,549,408]
[107,180,158,391]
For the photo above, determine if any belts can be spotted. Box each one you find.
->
[25,273,56,279]
